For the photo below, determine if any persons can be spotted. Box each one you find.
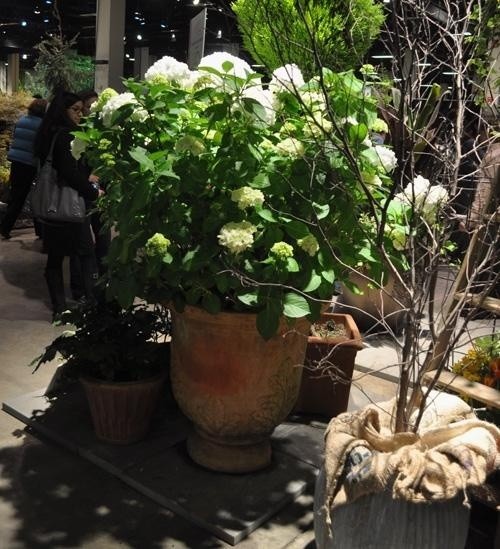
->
[0,98,51,240]
[29,91,106,324]
[73,90,115,304]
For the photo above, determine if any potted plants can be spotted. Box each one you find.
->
[158,268,500,549]
[27,265,173,447]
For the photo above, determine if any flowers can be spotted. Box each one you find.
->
[67,47,452,345]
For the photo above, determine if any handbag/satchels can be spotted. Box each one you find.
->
[30,162,86,222]
